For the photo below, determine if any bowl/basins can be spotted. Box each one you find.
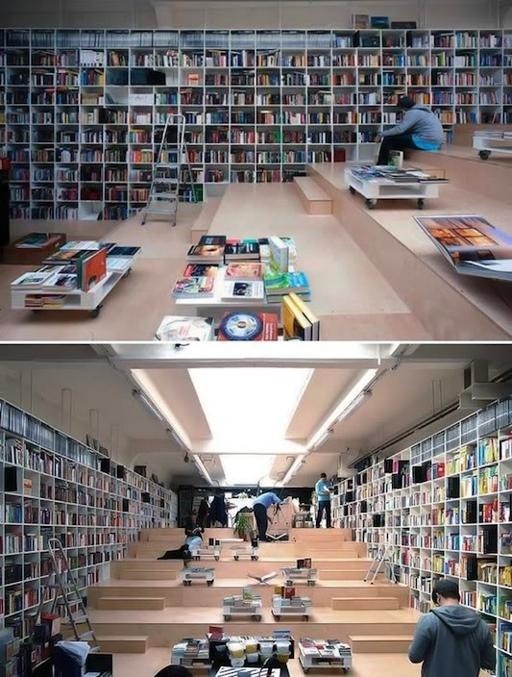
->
[227,640,291,667]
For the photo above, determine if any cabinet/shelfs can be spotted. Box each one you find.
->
[317,399,512,677]
[1,24,510,222]
[0,396,178,676]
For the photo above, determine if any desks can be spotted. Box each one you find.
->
[12,245,135,318]
[345,165,449,210]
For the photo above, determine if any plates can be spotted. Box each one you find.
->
[221,310,262,340]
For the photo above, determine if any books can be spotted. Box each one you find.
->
[1,32,511,220]
[154,235,320,341]
[412,215,512,282]
[1,398,512,676]
[10,236,142,308]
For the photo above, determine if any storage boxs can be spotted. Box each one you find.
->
[226,639,291,668]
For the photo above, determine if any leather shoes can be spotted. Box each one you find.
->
[316,524,320,528]
[327,524,334,528]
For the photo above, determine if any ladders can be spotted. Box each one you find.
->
[364,543,387,584]
[141,113,198,226]
[30,538,101,653]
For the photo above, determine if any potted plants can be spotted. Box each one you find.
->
[233,514,252,541]
